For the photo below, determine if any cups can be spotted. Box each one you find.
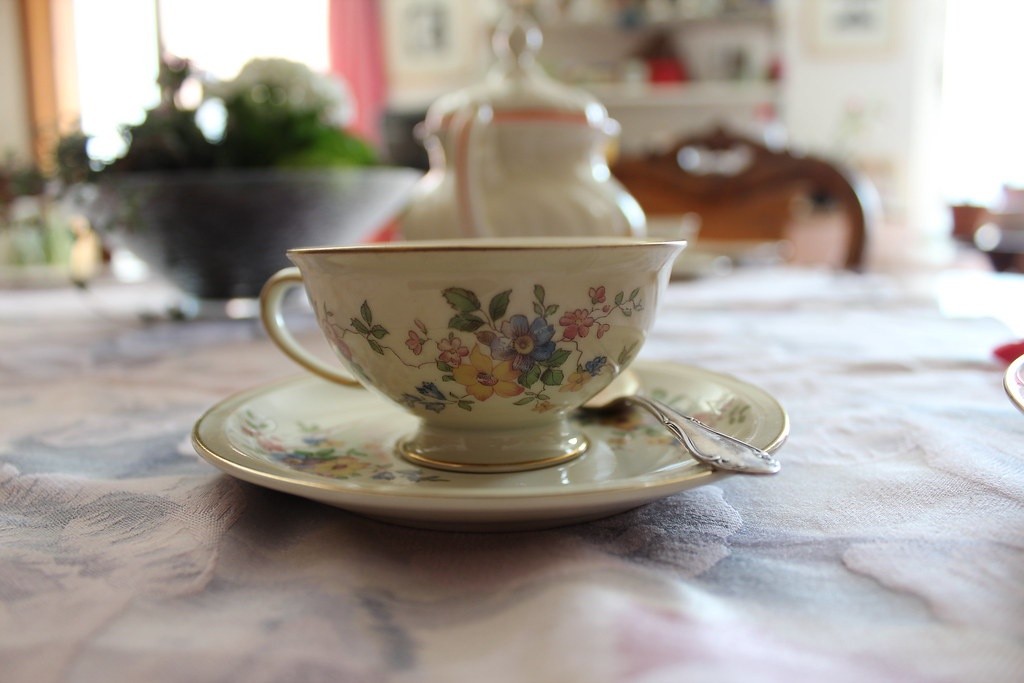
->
[259,241,689,472]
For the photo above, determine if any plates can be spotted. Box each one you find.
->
[1003,354,1024,413]
[191,360,790,529]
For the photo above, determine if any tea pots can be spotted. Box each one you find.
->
[390,18,647,236]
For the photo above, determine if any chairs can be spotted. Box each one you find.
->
[611,125,866,273]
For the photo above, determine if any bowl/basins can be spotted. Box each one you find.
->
[50,167,423,319]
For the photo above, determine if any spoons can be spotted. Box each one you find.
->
[583,370,781,474]
